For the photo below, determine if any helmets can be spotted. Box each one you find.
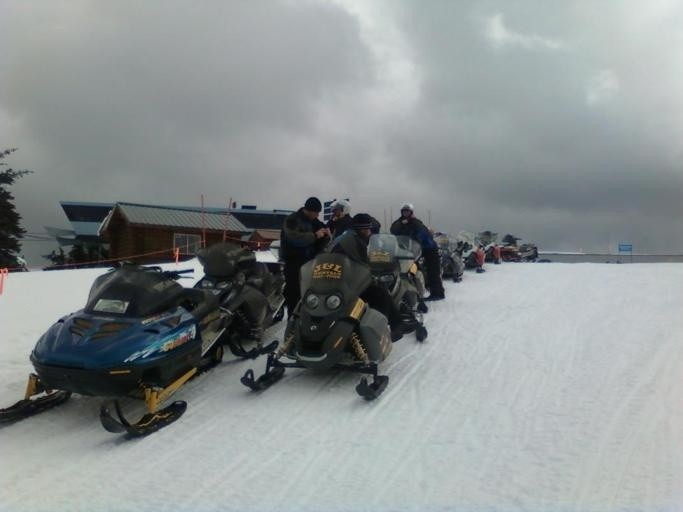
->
[401,204,414,217]
[330,200,351,221]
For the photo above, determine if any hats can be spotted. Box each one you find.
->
[305,197,321,212]
[352,214,373,228]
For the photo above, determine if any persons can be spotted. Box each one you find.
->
[406,223,445,300]
[281,198,332,319]
[390,204,423,235]
[325,199,353,238]
[329,212,400,332]
